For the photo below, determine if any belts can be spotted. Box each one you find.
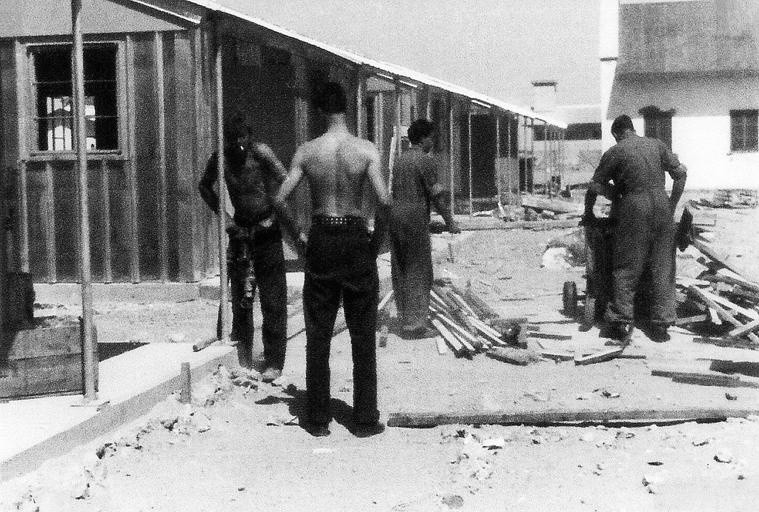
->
[312,217,365,226]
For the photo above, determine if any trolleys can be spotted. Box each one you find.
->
[562,207,619,327]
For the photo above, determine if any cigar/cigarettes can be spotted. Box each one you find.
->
[240,145,245,151]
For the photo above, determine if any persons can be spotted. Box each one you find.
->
[389,118,462,339]
[270,80,395,435]
[197,110,295,383]
[584,112,687,343]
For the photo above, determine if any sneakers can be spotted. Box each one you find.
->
[616,322,630,338]
[300,415,328,436]
[356,420,384,437]
[263,366,282,380]
[404,327,438,340]
[653,326,669,342]
